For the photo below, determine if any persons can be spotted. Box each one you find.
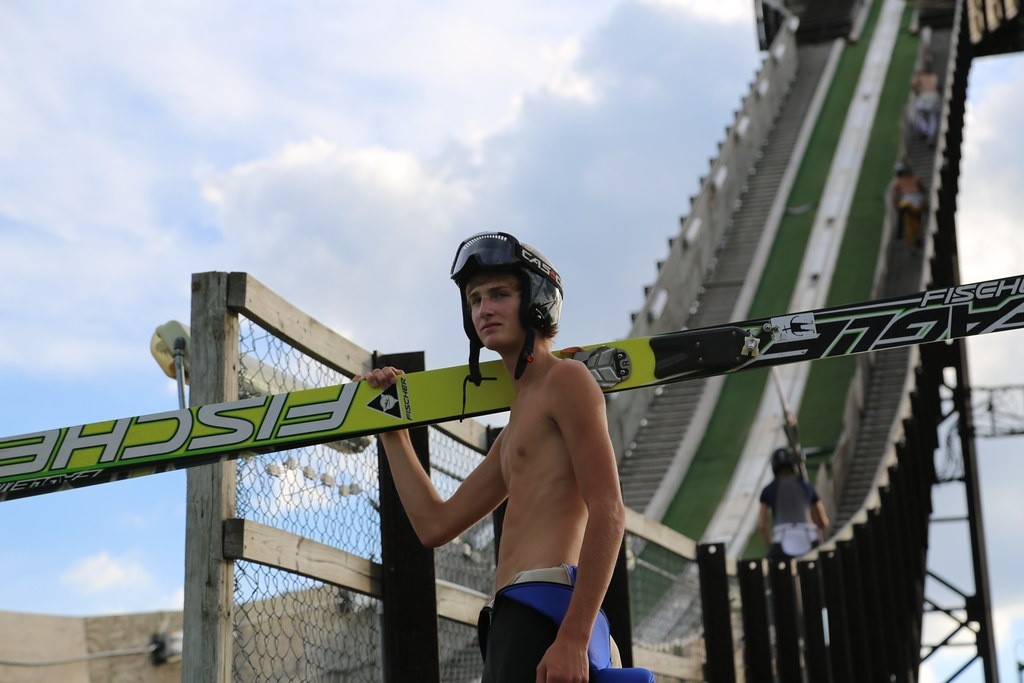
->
[892,163,932,256]
[759,448,830,669]
[912,56,943,145]
[353,233,626,683]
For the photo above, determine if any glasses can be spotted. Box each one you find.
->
[450,231,522,283]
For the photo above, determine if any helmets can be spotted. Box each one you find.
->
[455,241,562,335]
